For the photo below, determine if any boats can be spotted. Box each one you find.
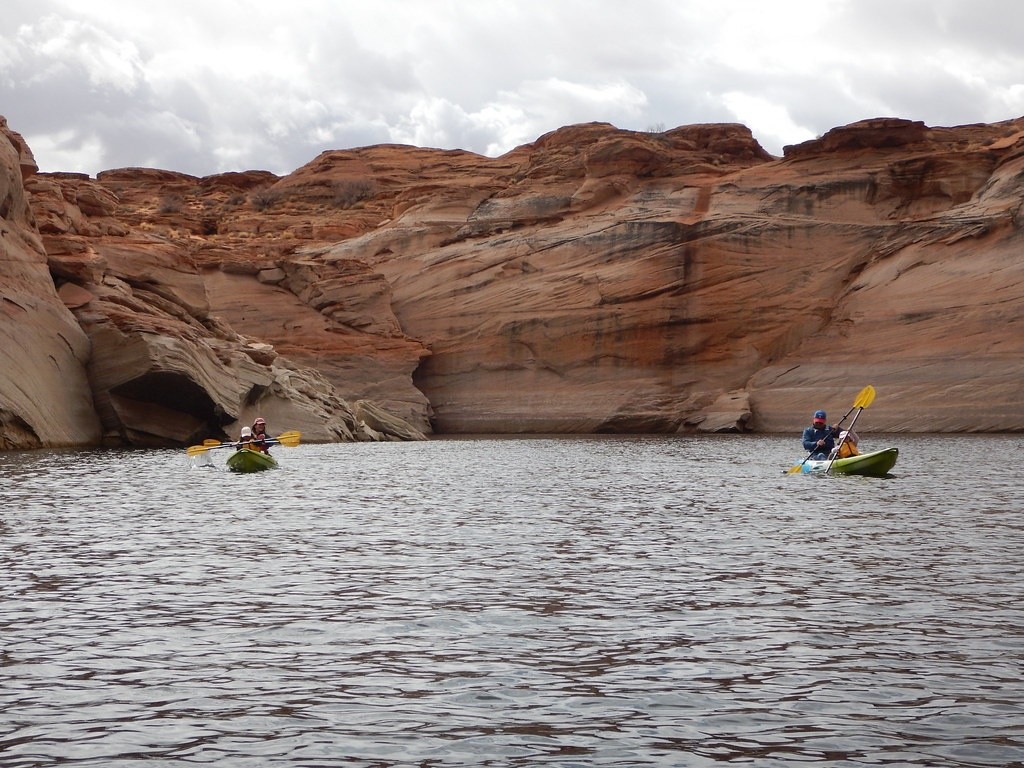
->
[226,447,278,473]
[796,447,898,478]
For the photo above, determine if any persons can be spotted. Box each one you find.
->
[236,418,273,453]
[802,410,845,460]
[832,427,860,458]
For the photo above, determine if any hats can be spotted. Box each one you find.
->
[254,417,266,424]
[241,426,251,437]
[839,430,850,439]
[814,410,826,418]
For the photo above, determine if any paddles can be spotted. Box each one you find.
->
[186,430,302,455]
[787,384,875,473]
